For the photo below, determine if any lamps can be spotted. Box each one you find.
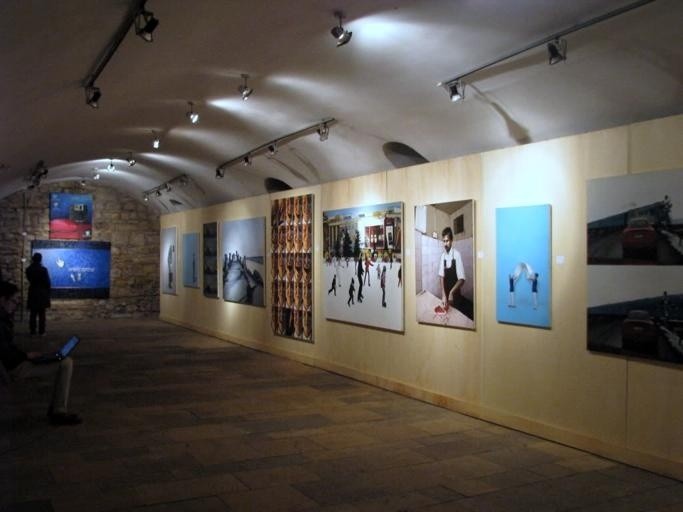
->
[81,0,161,109]
[238,74,256,101]
[186,101,201,126]
[450,37,567,103]
[216,118,338,178]
[25,160,49,190]
[151,130,161,153]
[330,11,353,48]
[127,152,136,168]
[143,174,189,202]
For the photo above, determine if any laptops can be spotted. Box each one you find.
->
[31,335,81,365]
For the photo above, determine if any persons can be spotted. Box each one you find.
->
[508,274,518,308]
[438,227,466,309]
[223,251,246,272]
[0,283,58,369]
[25,253,52,336]
[325,249,401,309]
[271,196,311,343]
[527,273,538,309]
